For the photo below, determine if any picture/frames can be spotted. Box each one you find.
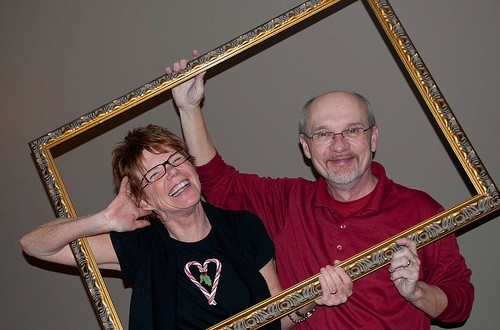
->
[29,0,499,330]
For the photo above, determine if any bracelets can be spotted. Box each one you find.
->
[287,314,308,324]
[295,308,316,318]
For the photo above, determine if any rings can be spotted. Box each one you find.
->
[405,260,411,268]
[127,192,131,195]
[330,290,337,295]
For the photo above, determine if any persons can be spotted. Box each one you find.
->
[163,48,474,330]
[18,124,353,330]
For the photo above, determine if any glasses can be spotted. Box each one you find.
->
[303,123,374,141]
[139,149,191,193]
